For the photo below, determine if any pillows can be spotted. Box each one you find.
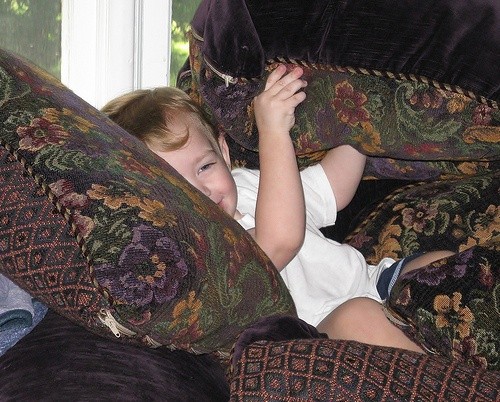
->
[342,173,500,266]
[188,1,499,160]
[0,46,297,358]
[230,315,500,402]
[382,232,500,363]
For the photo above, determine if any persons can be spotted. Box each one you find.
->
[97,64,457,356]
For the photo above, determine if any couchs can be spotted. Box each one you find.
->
[0,58,408,402]
